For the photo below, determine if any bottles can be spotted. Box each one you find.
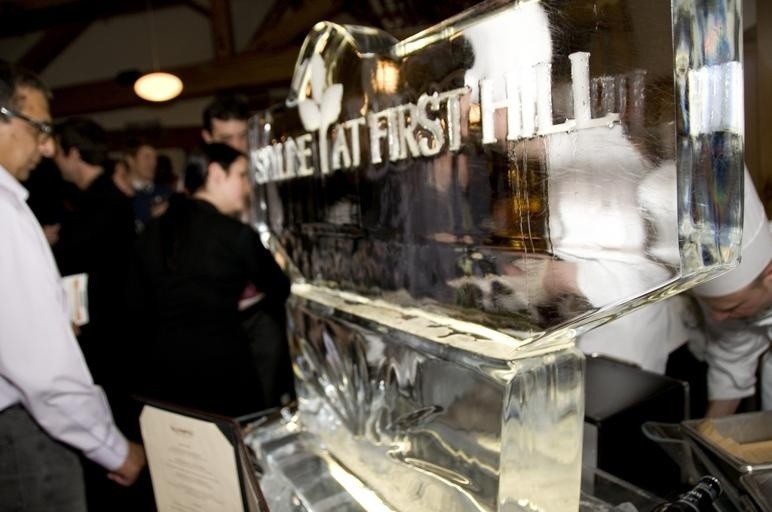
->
[650,477,723,512]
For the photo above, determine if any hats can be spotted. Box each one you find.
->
[636,159,772,299]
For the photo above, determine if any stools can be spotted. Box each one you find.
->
[584,345,710,494]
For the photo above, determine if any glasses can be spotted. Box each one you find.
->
[0,107,54,135]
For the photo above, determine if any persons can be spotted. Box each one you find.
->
[295,37,492,302]
[689,253,772,418]
[455,88,690,380]
[13,90,295,422]
[2,57,147,512]
